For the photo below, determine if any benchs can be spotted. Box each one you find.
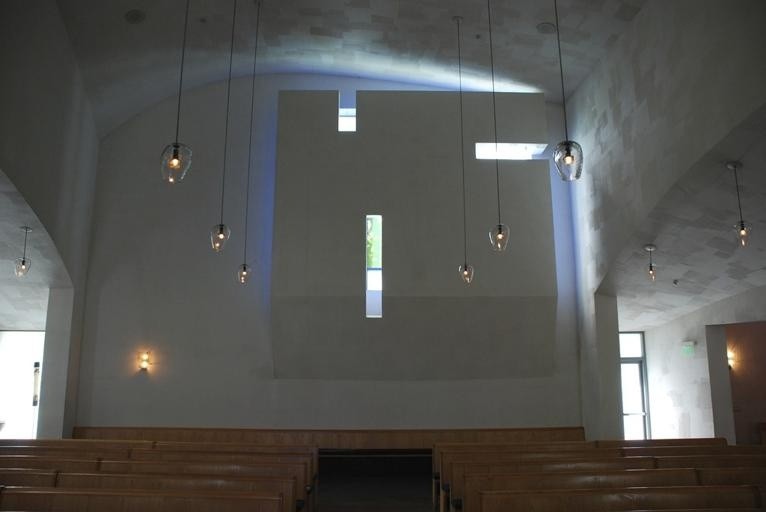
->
[433,435,766,512]
[1,433,322,511]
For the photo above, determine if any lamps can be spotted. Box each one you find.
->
[643,243,659,283]
[11,224,37,278]
[726,159,753,249]
[157,1,261,287]
[456,2,585,286]
